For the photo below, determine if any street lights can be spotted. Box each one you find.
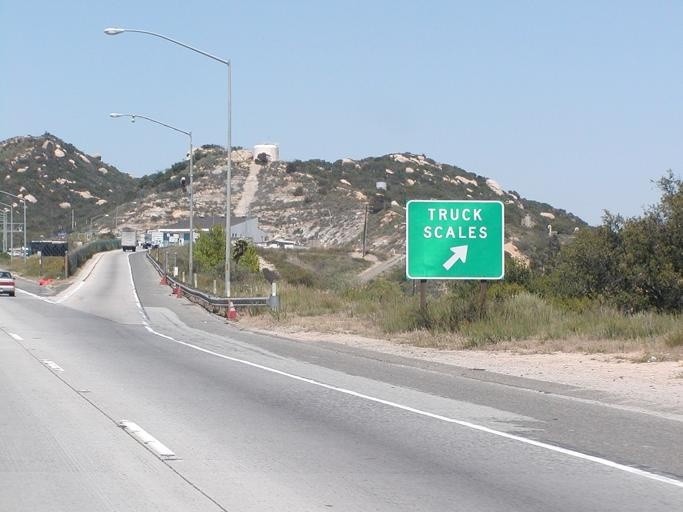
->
[102,24,234,306]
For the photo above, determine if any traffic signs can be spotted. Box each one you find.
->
[403,197,507,282]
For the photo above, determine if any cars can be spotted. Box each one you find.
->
[0,270,17,296]
